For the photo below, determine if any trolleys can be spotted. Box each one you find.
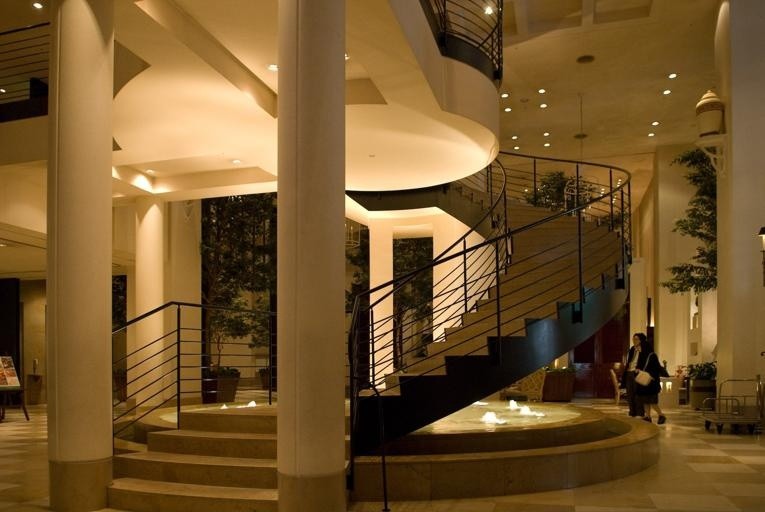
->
[700,379,761,434]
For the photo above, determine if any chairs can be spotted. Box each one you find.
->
[504,367,547,401]
[610,368,627,406]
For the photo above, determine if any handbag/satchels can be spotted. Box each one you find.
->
[635,370,652,386]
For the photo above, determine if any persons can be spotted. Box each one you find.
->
[619,333,666,424]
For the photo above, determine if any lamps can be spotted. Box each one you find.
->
[694,88,729,180]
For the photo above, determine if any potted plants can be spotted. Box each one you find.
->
[201,194,276,403]
[688,361,716,409]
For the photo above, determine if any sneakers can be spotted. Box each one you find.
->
[641,417,652,423]
[658,414,666,424]
